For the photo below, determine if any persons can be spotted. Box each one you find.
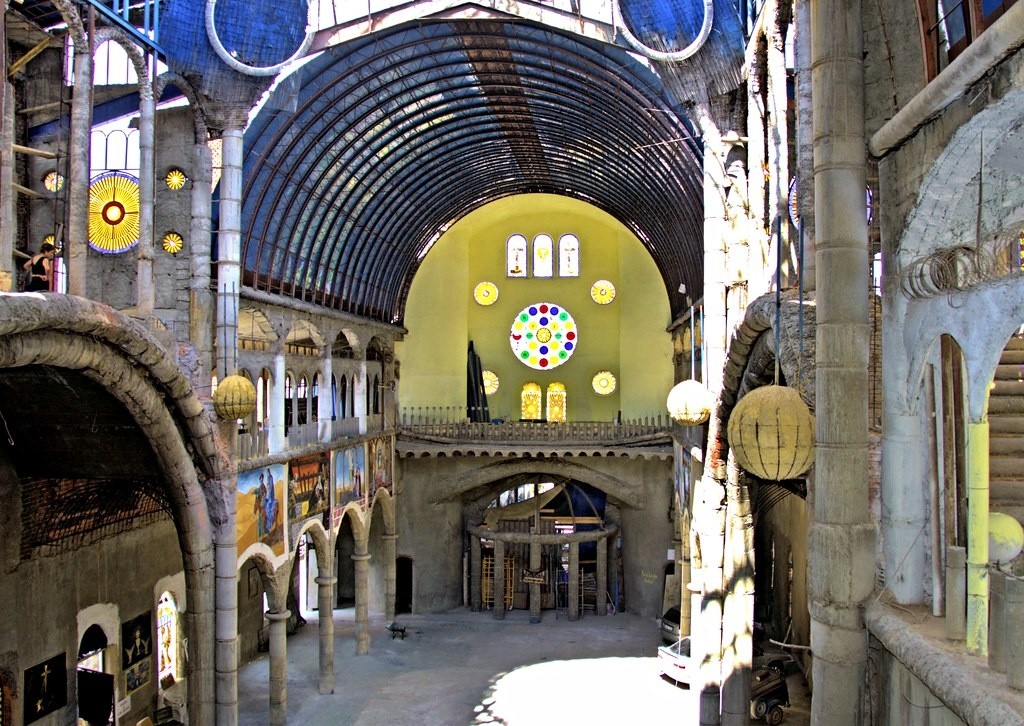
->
[23,242,54,293]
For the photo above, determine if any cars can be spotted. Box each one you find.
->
[658,636,793,685]
[661,605,764,643]
